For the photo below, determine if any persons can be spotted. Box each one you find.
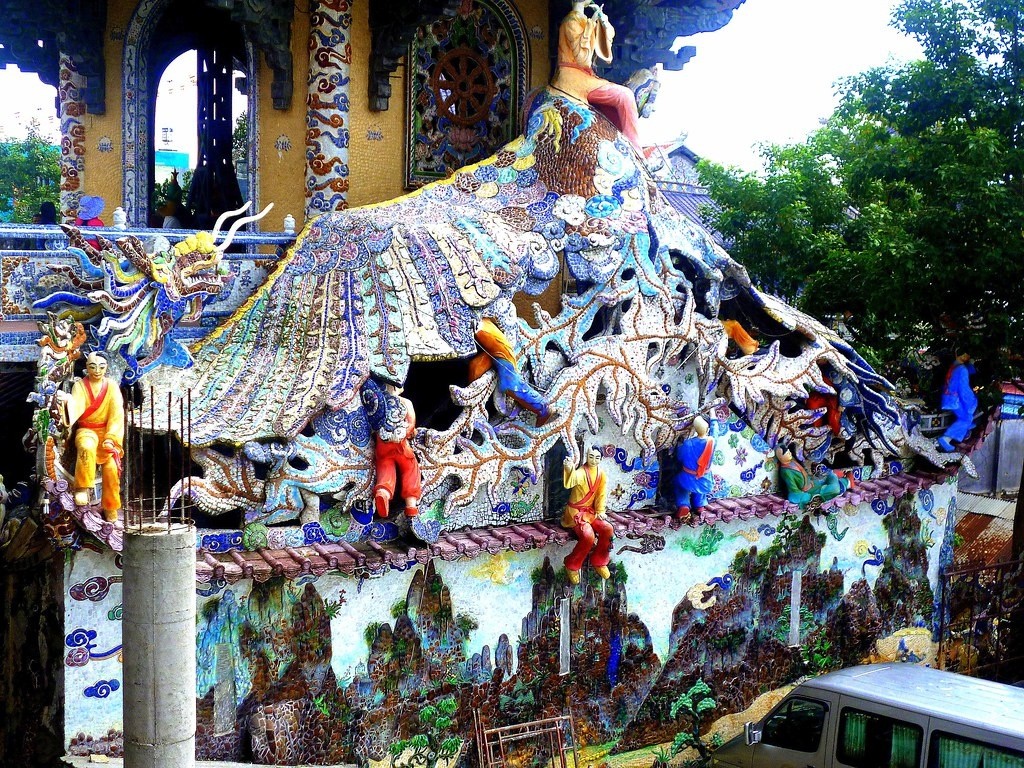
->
[719,317,770,356]
[370,382,421,517]
[801,372,847,436]
[57,350,125,524]
[25,196,230,250]
[671,407,718,517]
[469,318,557,427]
[776,445,856,502]
[561,442,613,584]
[552,1,643,151]
[937,343,978,452]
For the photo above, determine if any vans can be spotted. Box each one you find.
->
[707,663,1024,768]
